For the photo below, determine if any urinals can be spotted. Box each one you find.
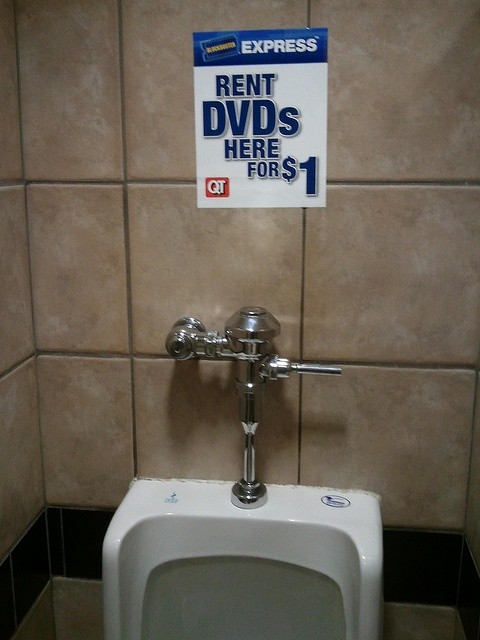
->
[102,480,383,640]
